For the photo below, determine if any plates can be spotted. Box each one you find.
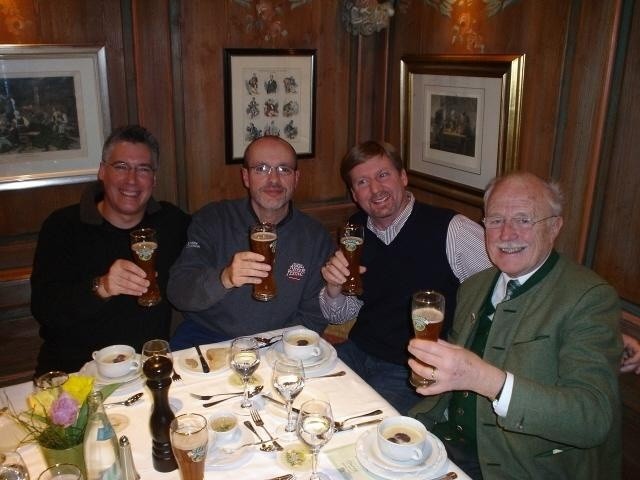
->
[265,337,338,374]
[204,422,255,470]
[80,353,149,392]
[355,427,448,479]
[364,427,440,473]
[177,344,232,376]
[273,342,331,368]
[83,360,145,385]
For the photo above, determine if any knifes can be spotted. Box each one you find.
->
[260,394,310,418]
[195,345,210,373]
[333,418,384,433]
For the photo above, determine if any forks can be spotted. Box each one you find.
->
[189,392,251,401]
[243,420,276,452]
[250,409,284,451]
[171,367,184,383]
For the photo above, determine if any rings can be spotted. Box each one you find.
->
[431,369,435,379]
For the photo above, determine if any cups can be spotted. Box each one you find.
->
[169,413,209,480]
[229,335,261,416]
[282,329,322,361]
[37,464,85,480]
[273,357,305,442]
[251,222,278,301]
[207,411,240,442]
[92,345,141,378]
[1,449,30,480]
[410,291,446,388]
[377,416,427,461]
[32,370,70,397]
[339,219,365,296]
[130,226,162,307]
[297,399,336,480]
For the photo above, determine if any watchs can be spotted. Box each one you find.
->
[90,272,102,302]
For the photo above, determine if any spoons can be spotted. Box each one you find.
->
[102,392,144,407]
[332,409,383,430]
[203,384,264,408]
[254,334,282,344]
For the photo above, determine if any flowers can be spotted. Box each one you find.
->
[0,372,125,448]
[341,0,396,37]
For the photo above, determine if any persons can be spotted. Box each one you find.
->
[436,105,469,134]
[320,140,638,419]
[245,73,298,138]
[169,136,334,351]
[29,126,189,379]
[10,105,68,150]
[407,171,622,476]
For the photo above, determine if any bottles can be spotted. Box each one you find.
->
[84,392,120,480]
[117,436,139,480]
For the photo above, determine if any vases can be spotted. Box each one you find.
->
[35,439,89,480]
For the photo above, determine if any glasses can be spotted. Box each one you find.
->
[245,165,294,176]
[102,159,155,176]
[483,215,559,229]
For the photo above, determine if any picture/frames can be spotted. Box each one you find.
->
[0,42,112,193]
[223,48,318,165]
[398,52,526,208]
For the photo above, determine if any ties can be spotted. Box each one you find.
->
[501,280,517,303]
